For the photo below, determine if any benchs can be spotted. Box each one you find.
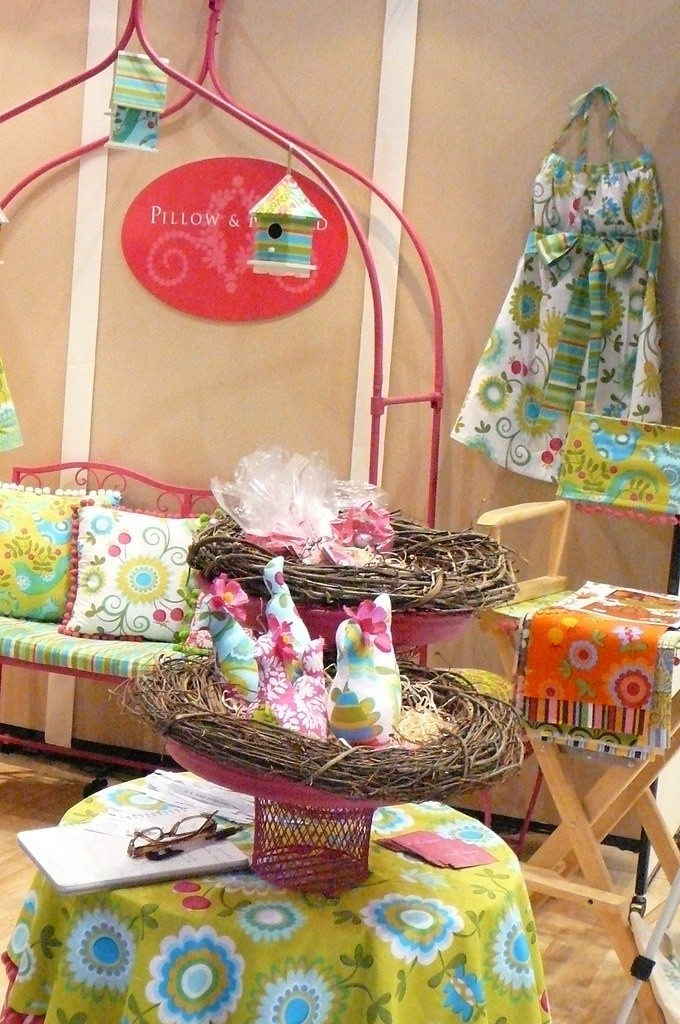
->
[0,461,220,773]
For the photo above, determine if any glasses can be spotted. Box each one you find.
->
[127,810,220,859]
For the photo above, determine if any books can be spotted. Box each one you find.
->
[16,807,249,895]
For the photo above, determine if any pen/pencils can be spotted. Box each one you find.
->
[145,823,251,861]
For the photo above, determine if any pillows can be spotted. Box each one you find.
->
[58,499,213,642]
[0,483,120,626]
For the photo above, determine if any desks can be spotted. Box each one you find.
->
[0,773,556,1024]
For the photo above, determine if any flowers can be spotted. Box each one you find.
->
[266,615,299,664]
[343,600,393,653]
[210,572,250,622]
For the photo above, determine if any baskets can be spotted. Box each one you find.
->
[191,566,474,673]
[165,706,476,895]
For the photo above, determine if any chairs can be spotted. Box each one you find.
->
[475,396,680,1024]
